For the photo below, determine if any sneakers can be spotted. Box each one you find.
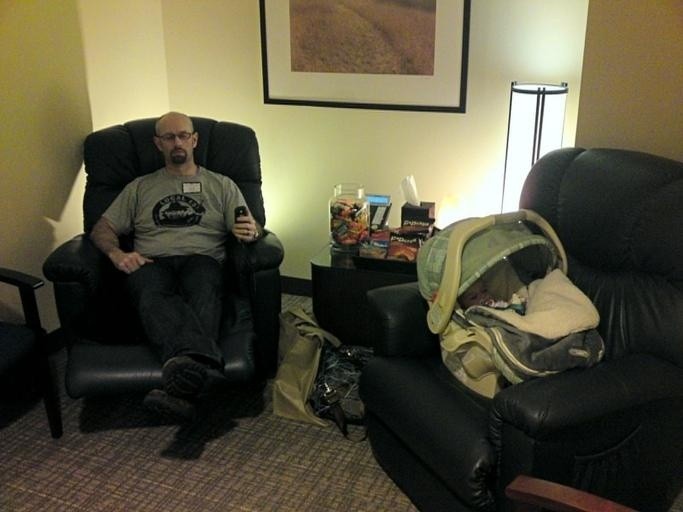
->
[159,356,235,405]
[141,387,212,428]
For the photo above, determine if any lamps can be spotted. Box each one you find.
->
[502,82,569,212]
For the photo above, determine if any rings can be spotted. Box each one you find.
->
[246,229,250,235]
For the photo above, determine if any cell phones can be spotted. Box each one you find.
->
[234,206,248,224]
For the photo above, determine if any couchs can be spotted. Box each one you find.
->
[43,117,285,434]
[359,147,683,511]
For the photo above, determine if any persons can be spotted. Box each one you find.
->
[459,280,527,317]
[89,111,261,416]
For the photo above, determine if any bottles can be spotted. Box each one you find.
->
[328,182,370,252]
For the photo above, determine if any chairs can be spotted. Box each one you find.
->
[1,268,64,440]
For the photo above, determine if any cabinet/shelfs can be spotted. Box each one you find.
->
[310,236,416,349]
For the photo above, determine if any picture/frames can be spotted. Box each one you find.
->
[259,0,471,115]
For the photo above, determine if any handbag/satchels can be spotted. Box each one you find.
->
[271,304,342,428]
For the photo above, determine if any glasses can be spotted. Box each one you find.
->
[154,132,194,142]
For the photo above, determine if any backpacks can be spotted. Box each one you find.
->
[314,343,372,427]
